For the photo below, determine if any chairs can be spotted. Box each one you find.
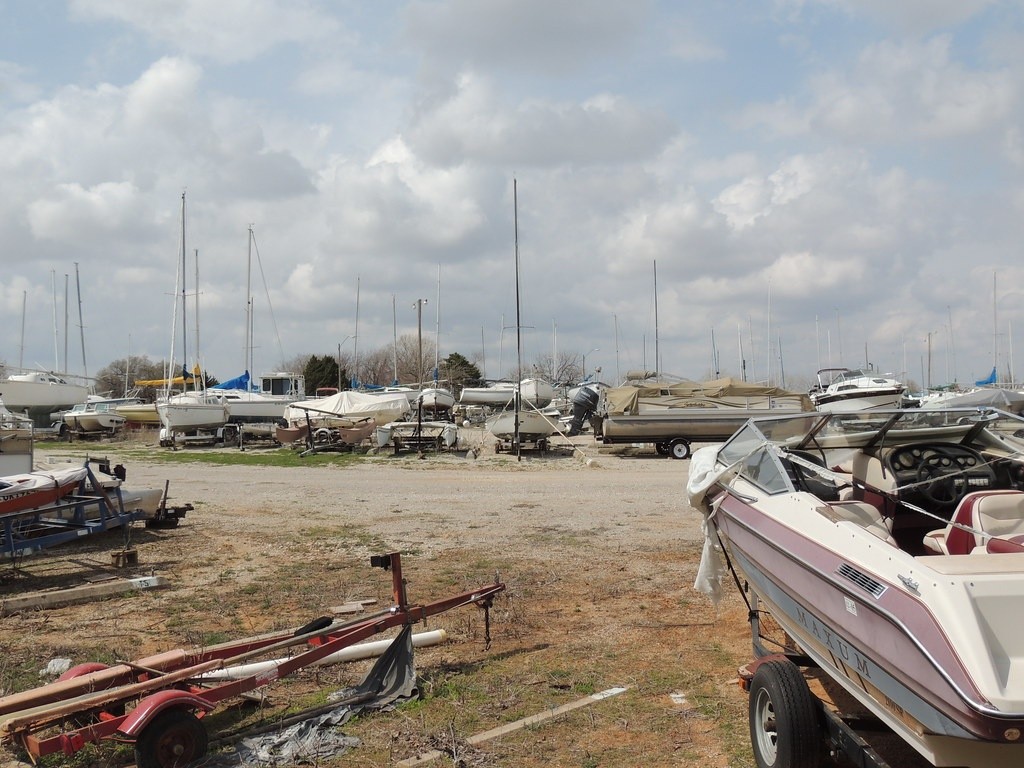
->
[922,490,1024,555]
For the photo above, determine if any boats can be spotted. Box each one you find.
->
[14,490,164,519]
[686,403,1024,745]
[0,466,88,515]
[809,368,909,414]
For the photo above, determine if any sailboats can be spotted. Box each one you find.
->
[0,178,1024,459]
